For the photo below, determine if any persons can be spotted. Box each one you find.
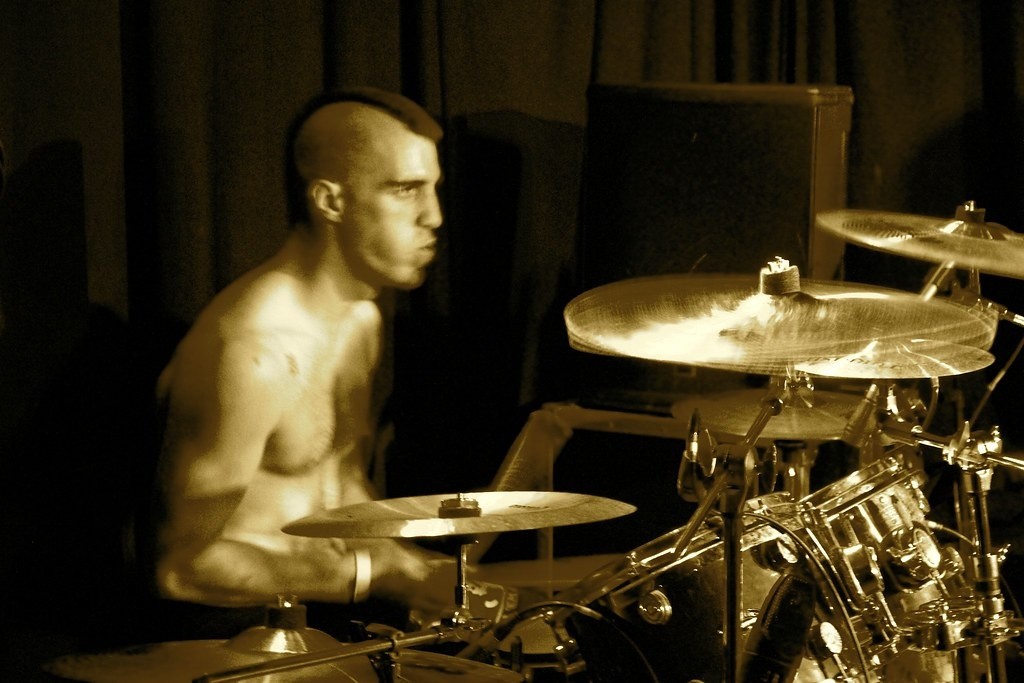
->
[156,86,459,606]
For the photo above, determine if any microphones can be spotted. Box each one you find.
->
[676,409,710,502]
[843,380,896,449]
[736,567,817,683]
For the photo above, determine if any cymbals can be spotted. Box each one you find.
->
[48,626,526,683]
[795,335,996,380]
[281,490,637,539]
[669,389,883,441]
[560,272,998,375]
[814,208,1024,279]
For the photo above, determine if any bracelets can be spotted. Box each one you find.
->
[352,548,372,603]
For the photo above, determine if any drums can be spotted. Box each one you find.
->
[551,489,878,683]
[796,441,982,653]
